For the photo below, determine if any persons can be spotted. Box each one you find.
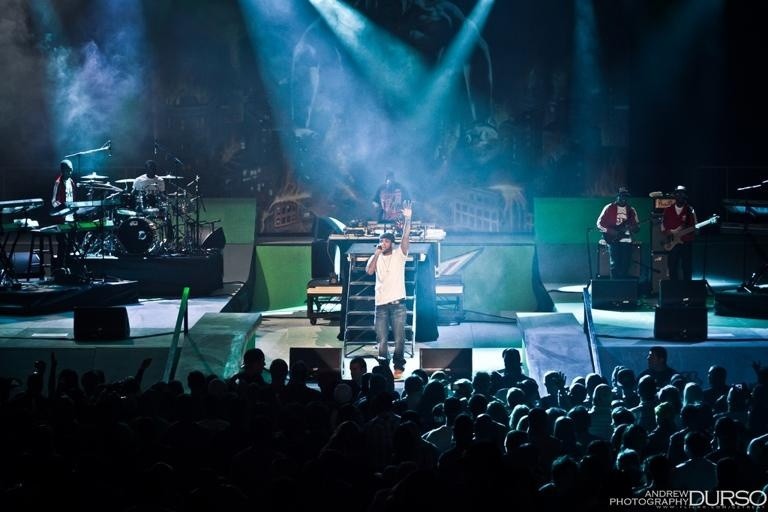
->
[131,160,175,254]
[596,185,640,280]
[660,186,700,280]
[372,173,416,224]
[365,199,413,379]
[0,346,768,512]
[52,160,74,265]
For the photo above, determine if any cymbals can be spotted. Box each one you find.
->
[115,179,135,183]
[81,181,103,183]
[161,175,183,179]
[80,175,109,179]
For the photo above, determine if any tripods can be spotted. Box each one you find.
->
[153,162,206,253]
[703,234,714,297]
[719,221,755,294]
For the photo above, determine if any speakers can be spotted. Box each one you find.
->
[289,347,347,381]
[658,279,705,306]
[73,304,131,342]
[418,347,474,381]
[654,306,708,343]
[5,250,46,281]
[714,292,768,320]
[586,277,637,310]
[192,225,226,249]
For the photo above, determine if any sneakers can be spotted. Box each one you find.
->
[393,369,402,380]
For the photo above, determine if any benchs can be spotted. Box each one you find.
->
[306,284,465,325]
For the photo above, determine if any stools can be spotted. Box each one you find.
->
[26,229,58,282]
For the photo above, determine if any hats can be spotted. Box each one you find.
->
[616,186,633,198]
[673,184,688,193]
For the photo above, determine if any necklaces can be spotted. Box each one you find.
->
[382,255,392,273]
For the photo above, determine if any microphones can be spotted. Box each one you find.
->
[175,157,183,166]
[107,141,112,159]
[377,245,382,249]
[152,137,158,156]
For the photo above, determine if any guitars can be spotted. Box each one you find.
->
[661,214,719,251]
[603,217,663,246]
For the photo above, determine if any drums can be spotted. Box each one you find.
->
[104,193,121,210]
[172,195,192,214]
[142,189,159,212]
[115,193,136,216]
[117,216,157,256]
